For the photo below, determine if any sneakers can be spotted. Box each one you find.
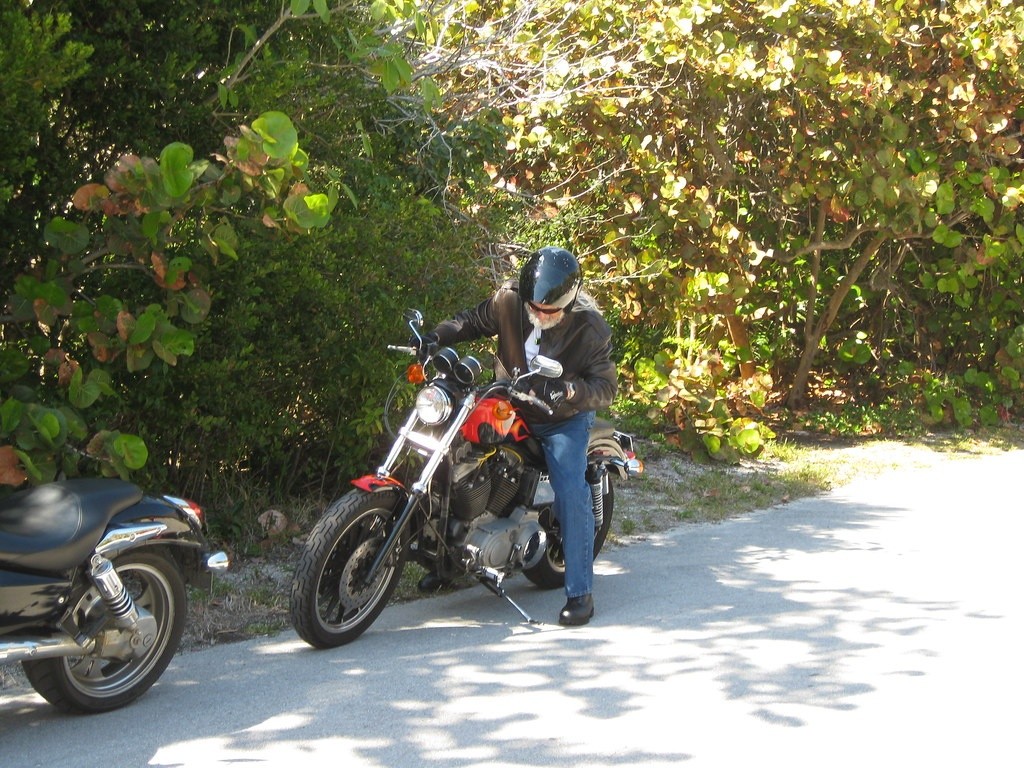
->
[559,594,595,625]
[418,572,443,593]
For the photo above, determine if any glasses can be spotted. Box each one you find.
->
[528,301,562,314]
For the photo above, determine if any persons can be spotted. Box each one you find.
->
[406,243,620,628]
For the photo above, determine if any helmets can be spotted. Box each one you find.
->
[518,246,582,314]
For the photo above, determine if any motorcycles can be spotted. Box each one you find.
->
[1,476,230,716]
[288,306,644,651]
